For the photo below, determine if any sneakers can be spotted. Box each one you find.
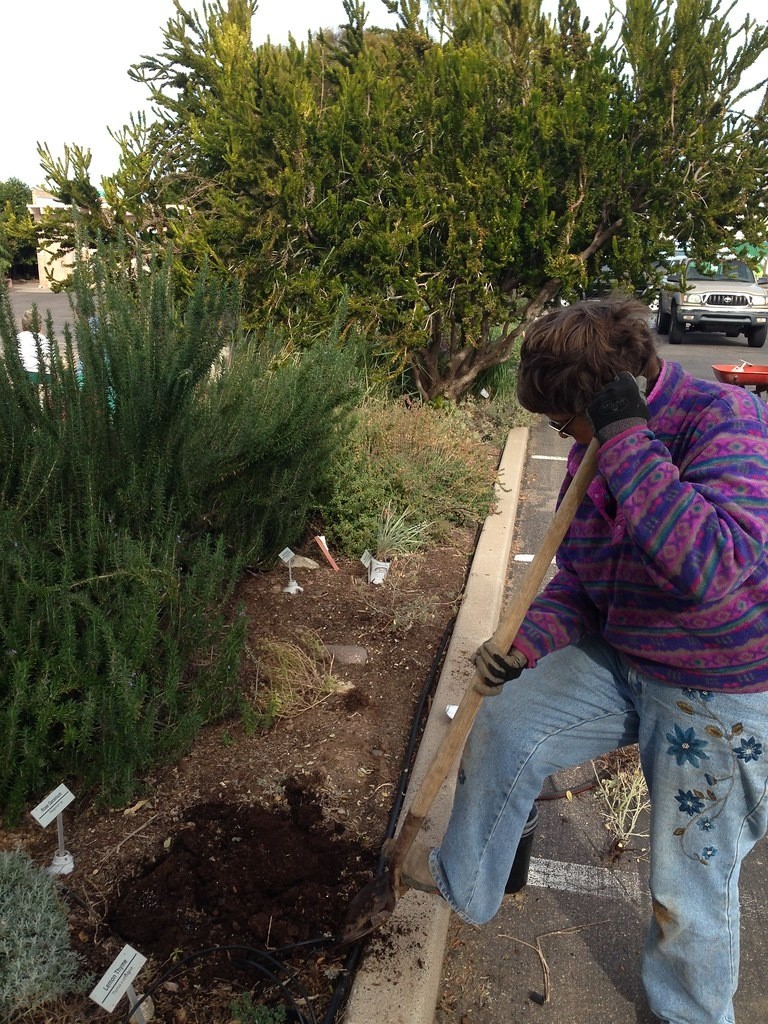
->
[384,836,443,897]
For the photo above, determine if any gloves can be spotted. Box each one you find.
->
[473,636,527,695]
[586,371,647,439]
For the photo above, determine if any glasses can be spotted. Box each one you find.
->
[546,411,580,437]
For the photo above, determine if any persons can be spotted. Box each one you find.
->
[382,296,768,1023]
[72,298,115,421]
[207,318,236,383]
[16,308,58,414]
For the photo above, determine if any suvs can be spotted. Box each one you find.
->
[597,240,767,350]
